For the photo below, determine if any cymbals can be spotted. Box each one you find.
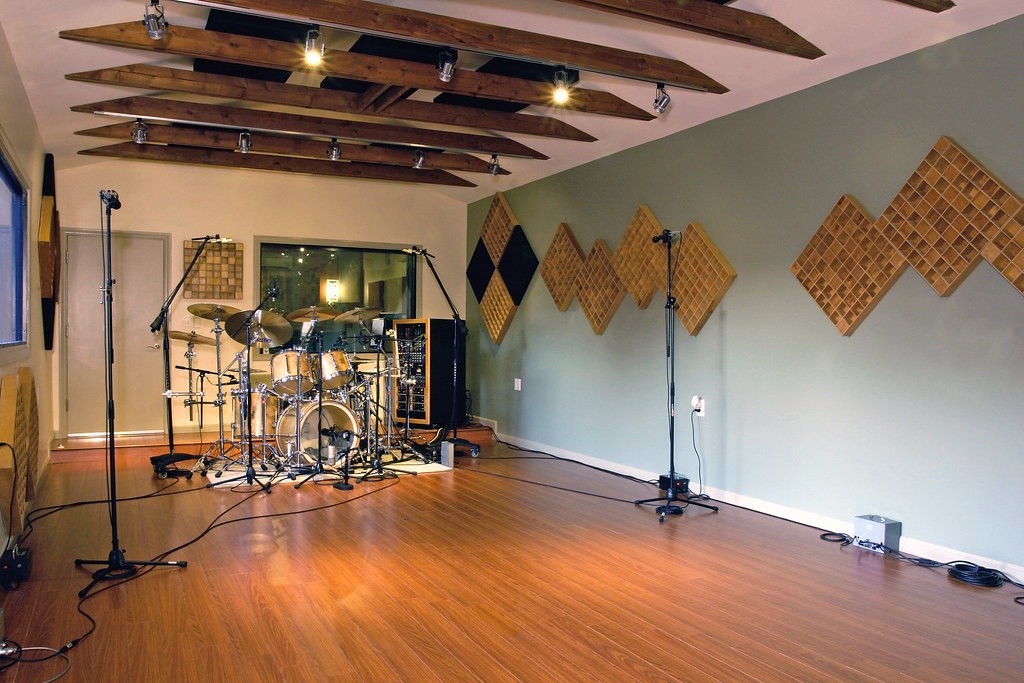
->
[187,304,243,322]
[334,306,385,323]
[156,330,222,346]
[286,307,340,322]
[227,366,268,374]
[348,357,374,364]
[225,309,293,348]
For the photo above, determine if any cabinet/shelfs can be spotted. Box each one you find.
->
[393,318,466,428]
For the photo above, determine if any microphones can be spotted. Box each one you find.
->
[271,284,277,302]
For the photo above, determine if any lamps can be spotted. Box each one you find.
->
[653,83,670,113]
[304,24,324,65]
[131,118,148,143]
[439,49,457,82]
[553,65,569,104]
[488,154,500,176]
[236,130,252,153]
[326,138,341,160]
[412,149,424,169]
[142,0,168,41]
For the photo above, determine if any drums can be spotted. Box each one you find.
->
[270,347,316,396]
[310,348,355,392]
[275,400,360,469]
[229,388,278,438]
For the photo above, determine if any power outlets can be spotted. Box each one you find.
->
[514,378,522,391]
[695,399,705,416]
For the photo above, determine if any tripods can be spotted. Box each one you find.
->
[74,208,188,599]
[187,318,459,494]
[635,240,718,522]
